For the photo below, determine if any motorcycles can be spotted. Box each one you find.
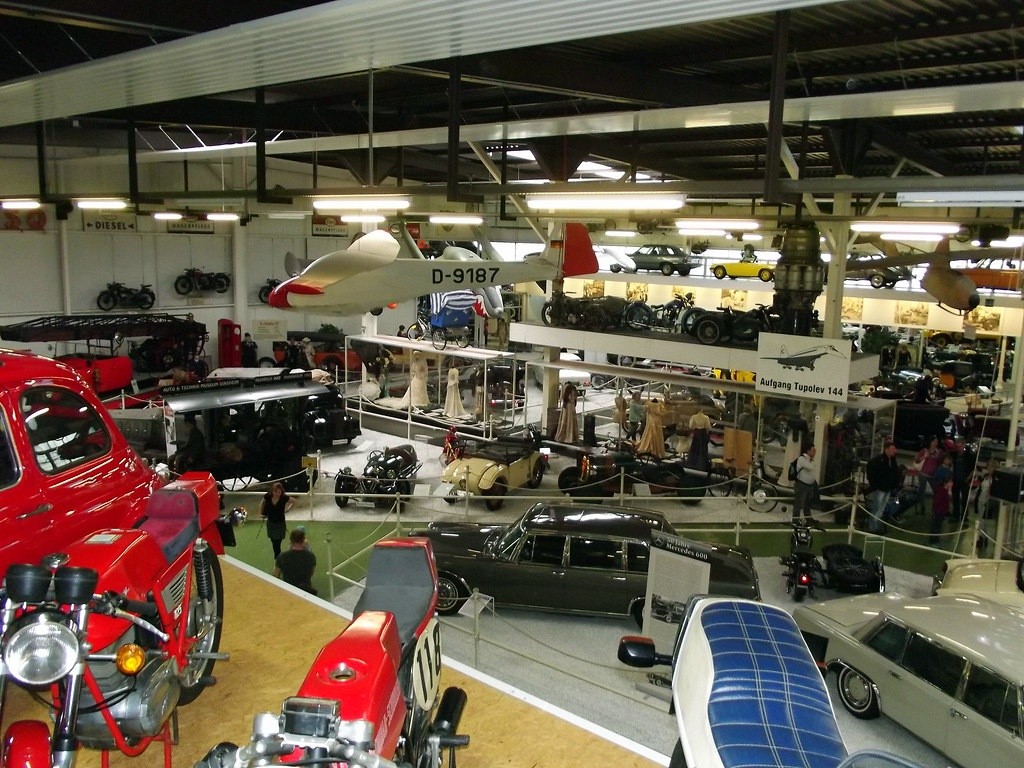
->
[0,473,237,768]
[258,278,283,305]
[190,534,471,767]
[624,291,711,336]
[689,298,781,348]
[96,281,157,311]
[778,519,826,603]
[174,265,231,295]
[618,594,926,768]
[541,289,607,333]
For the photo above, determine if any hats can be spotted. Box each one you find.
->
[245,333,250,337]
[302,337,311,342]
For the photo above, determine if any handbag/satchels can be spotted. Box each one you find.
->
[913,449,927,471]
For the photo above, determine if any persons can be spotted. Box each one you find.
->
[626,393,645,441]
[258,482,298,574]
[470,366,480,396]
[558,382,563,397]
[914,437,992,547]
[689,407,712,470]
[443,425,457,453]
[634,398,667,459]
[180,417,208,471]
[898,345,911,365]
[554,381,580,444]
[283,336,317,370]
[241,332,259,369]
[272,529,318,594]
[358,351,484,420]
[793,443,820,526]
[867,442,904,531]
[881,346,896,366]
[737,403,758,461]
[397,325,412,339]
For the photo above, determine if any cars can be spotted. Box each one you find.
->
[404,502,764,632]
[44,353,133,393]
[0,344,168,591]
[609,243,703,277]
[789,589,1024,768]
[441,428,550,510]
[824,251,916,290]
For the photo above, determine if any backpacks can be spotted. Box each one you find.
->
[788,455,807,481]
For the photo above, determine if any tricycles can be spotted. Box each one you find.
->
[406,288,492,350]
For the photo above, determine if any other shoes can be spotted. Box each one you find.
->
[948,518,961,524]
[930,543,940,548]
[962,517,969,527]
[792,518,803,528]
[890,515,906,523]
[632,440,638,445]
[804,519,820,527]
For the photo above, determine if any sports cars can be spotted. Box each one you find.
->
[709,255,778,282]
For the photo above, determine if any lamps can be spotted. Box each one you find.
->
[309,194,413,213]
[524,192,689,211]
[850,221,961,233]
[175,197,246,207]
[72,200,128,209]
[674,217,760,231]
[897,190,1024,209]
[432,214,485,226]
[269,214,306,220]
[1,200,41,209]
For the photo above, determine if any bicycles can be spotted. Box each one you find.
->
[706,451,779,514]
[601,430,662,478]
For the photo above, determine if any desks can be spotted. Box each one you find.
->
[897,404,951,442]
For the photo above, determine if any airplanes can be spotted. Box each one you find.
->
[264,220,600,317]
[843,234,1024,319]
